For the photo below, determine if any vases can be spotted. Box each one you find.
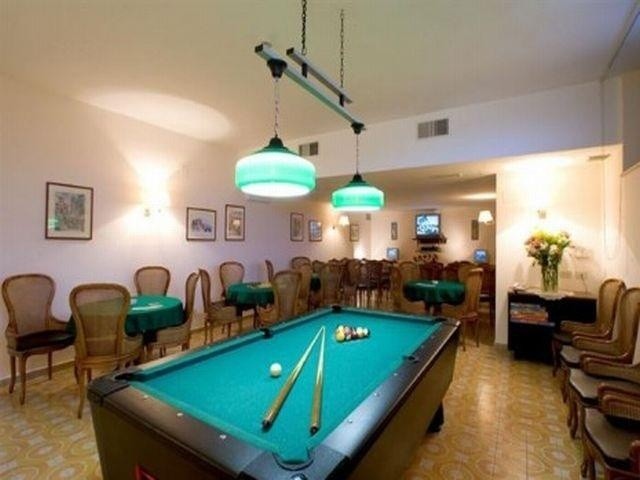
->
[542,265,558,291]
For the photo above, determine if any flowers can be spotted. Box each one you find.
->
[524,231,571,267]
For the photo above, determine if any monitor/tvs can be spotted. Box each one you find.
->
[415,215,440,239]
[474,250,490,264]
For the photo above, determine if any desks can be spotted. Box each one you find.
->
[89,305,462,480]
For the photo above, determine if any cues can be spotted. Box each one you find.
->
[262,326,325,434]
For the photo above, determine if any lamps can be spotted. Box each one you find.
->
[478,210,493,224]
[338,215,350,228]
[234,1,385,213]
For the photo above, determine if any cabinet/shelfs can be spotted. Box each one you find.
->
[508,286,597,366]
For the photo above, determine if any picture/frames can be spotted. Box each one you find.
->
[225,205,245,241]
[350,225,360,241]
[309,220,322,242]
[186,207,216,241]
[44,183,94,240]
[290,213,304,241]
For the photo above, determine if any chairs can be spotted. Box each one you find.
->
[439,268,483,352]
[219,262,245,288]
[264,257,483,305]
[134,266,171,297]
[69,283,143,419]
[199,268,236,346]
[551,279,640,480]
[3,273,78,405]
[257,270,301,326]
[145,272,201,361]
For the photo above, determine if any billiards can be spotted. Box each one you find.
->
[335,324,369,342]
